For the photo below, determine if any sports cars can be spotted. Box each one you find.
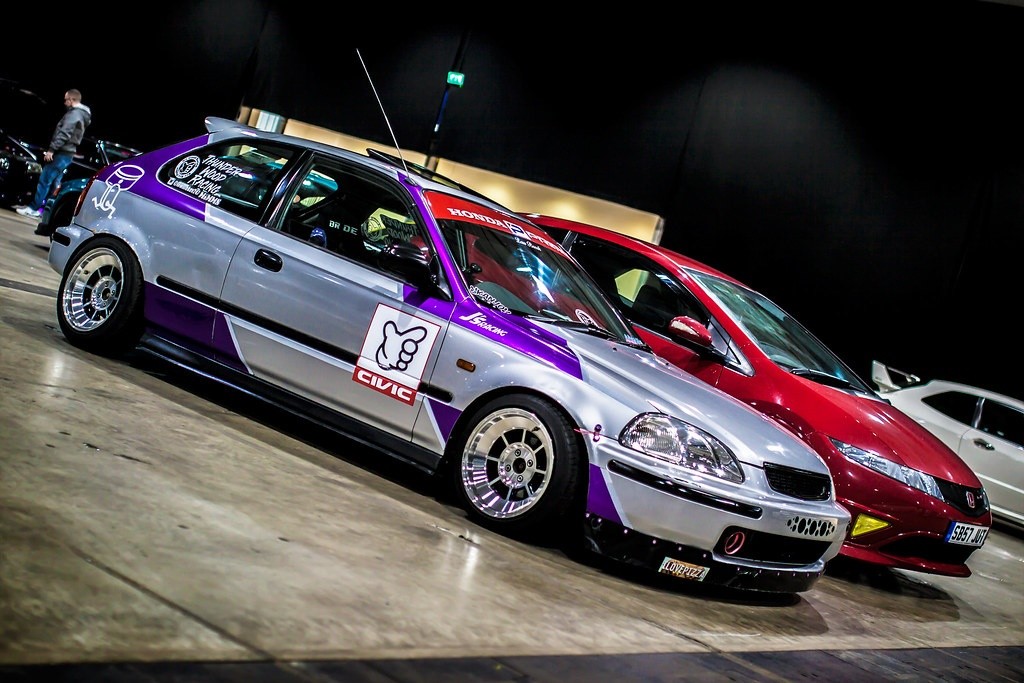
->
[867,359,1024,534]
[44,114,852,592]
[366,210,991,579]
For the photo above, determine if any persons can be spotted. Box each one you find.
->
[16,89,92,223]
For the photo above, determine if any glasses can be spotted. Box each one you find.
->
[64,98,71,102]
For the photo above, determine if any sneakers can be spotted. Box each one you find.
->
[16,206,39,220]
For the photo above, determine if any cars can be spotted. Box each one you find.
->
[0,77,144,208]
